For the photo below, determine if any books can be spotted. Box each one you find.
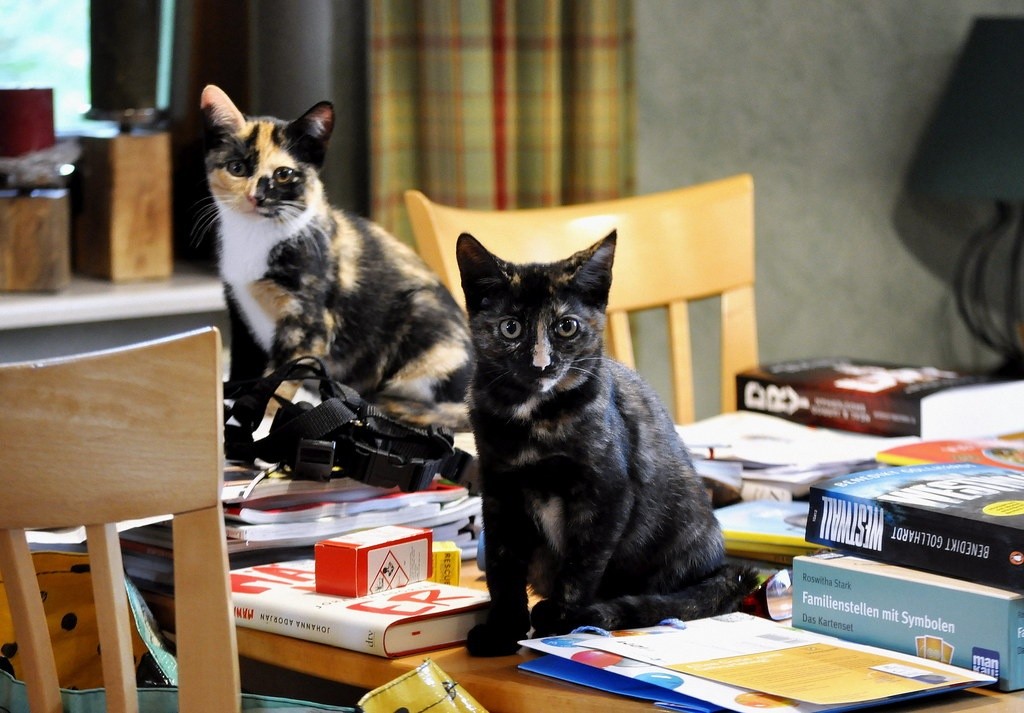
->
[24,460,490,658]
[669,356,1024,692]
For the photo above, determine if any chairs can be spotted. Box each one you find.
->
[0,324,243,713]
[402,170,759,426]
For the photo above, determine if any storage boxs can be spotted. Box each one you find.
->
[313,525,433,597]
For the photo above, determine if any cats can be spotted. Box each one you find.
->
[199,84,478,433]
[456,229,761,657]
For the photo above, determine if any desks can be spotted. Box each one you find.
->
[136,559,1024,713]
[0,261,232,363]
